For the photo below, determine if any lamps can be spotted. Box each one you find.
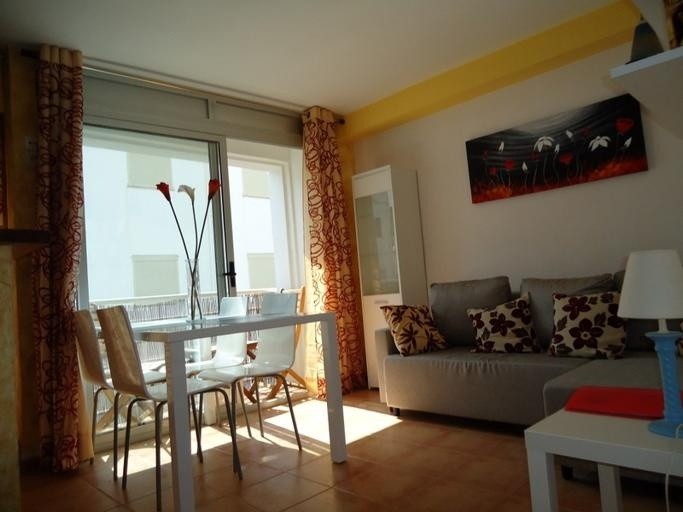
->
[615,251,682,436]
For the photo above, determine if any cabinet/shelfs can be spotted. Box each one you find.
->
[350,165,429,391]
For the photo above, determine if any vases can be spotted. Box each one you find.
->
[185,258,204,322]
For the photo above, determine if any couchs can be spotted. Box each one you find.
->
[374,273,682,490]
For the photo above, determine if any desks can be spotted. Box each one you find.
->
[523,405,682,512]
[97,311,347,509]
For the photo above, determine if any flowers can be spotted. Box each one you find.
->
[156,179,221,320]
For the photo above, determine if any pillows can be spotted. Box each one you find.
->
[466,291,542,355]
[545,292,635,359]
[380,306,450,358]
